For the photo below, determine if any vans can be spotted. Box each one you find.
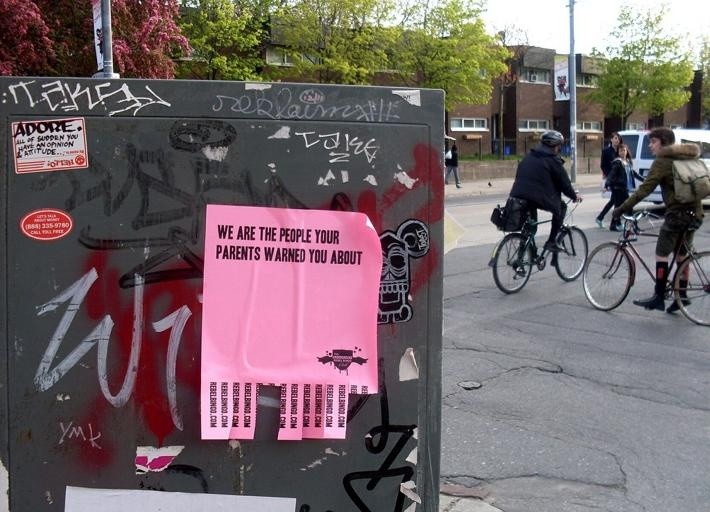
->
[600,128,710,207]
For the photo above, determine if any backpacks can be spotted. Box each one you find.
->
[671,159,710,204]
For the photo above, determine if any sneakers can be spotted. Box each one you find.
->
[611,224,625,232]
[595,217,603,228]
[666,298,691,313]
[543,240,564,251]
[633,295,665,310]
[513,261,526,274]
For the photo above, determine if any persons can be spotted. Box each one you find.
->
[445,144,463,188]
[612,129,704,313]
[604,145,645,232]
[596,134,629,230]
[513,130,583,277]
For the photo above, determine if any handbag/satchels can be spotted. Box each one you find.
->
[490,197,526,230]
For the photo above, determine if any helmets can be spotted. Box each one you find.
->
[540,130,565,147]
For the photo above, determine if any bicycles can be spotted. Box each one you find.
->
[488,190,588,294]
[582,207,710,326]
